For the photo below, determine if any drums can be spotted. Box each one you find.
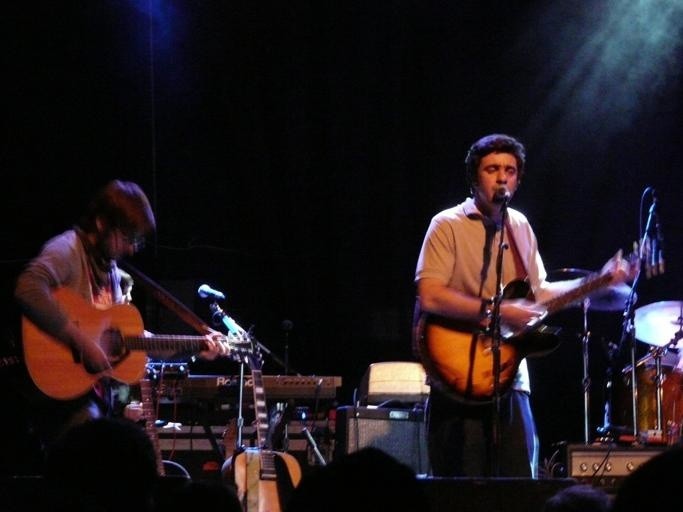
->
[635,365,683,446]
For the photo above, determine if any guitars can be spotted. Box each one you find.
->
[411,239,664,407]
[21,288,246,401]
[222,327,302,512]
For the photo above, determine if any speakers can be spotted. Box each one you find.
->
[335,406,434,476]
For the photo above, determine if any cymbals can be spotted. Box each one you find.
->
[627,301,683,351]
[546,268,637,313]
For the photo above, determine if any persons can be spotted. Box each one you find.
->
[8,182,232,462]
[411,133,641,479]
[36,412,683,512]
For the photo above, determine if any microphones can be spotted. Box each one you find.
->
[653,197,665,240]
[198,284,226,300]
[496,186,508,198]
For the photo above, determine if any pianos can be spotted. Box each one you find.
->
[158,370,343,404]
